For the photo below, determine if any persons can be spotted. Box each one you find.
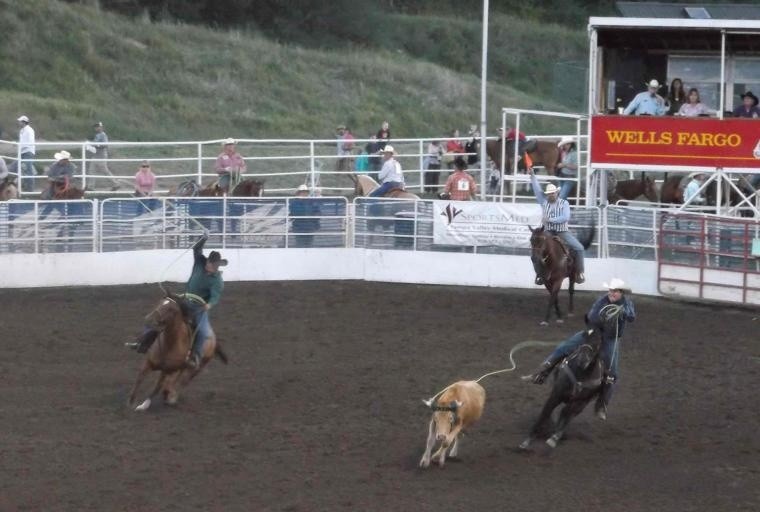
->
[288,184,321,248]
[620,79,760,118]
[45,150,77,199]
[212,137,247,189]
[7,115,36,192]
[526,167,585,286]
[334,120,579,200]
[440,157,475,253]
[682,172,709,246]
[132,161,156,216]
[124,235,228,408]
[84,121,122,191]
[520,278,636,420]
[0,158,9,187]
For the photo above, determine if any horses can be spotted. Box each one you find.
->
[124,288,228,416]
[516,314,607,454]
[465,138,563,200]
[347,171,425,250]
[166,180,266,243]
[527,220,598,328]
[0,178,18,253]
[660,175,757,231]
[36,177,88,252]
[551,170,658,214]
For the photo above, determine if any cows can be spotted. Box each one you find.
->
[419,381,487,468]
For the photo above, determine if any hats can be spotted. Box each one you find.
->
[741,92,758,106]
[381,146,394,155]
[222,138,235,144]
[689,172,704,178]
[54,151,70,160]
[200,251,227,266]
[453,159,466,170]
[558,137,575,147]
[17,116,29,122]
[544,184,561,194]
[295,185,308,193]
[603,279,629,290]
[94,121,102,126]
[645,79,662,88]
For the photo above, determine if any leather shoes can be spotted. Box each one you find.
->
[522,374,542,384]
[124,343,137,348]
[595,402,606,420]
